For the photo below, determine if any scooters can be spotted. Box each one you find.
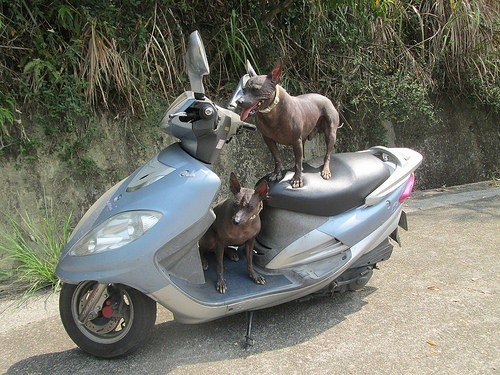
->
[53,31,425,359]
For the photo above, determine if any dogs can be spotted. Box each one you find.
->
[198,172,271,296]
[235,58,343,189]
